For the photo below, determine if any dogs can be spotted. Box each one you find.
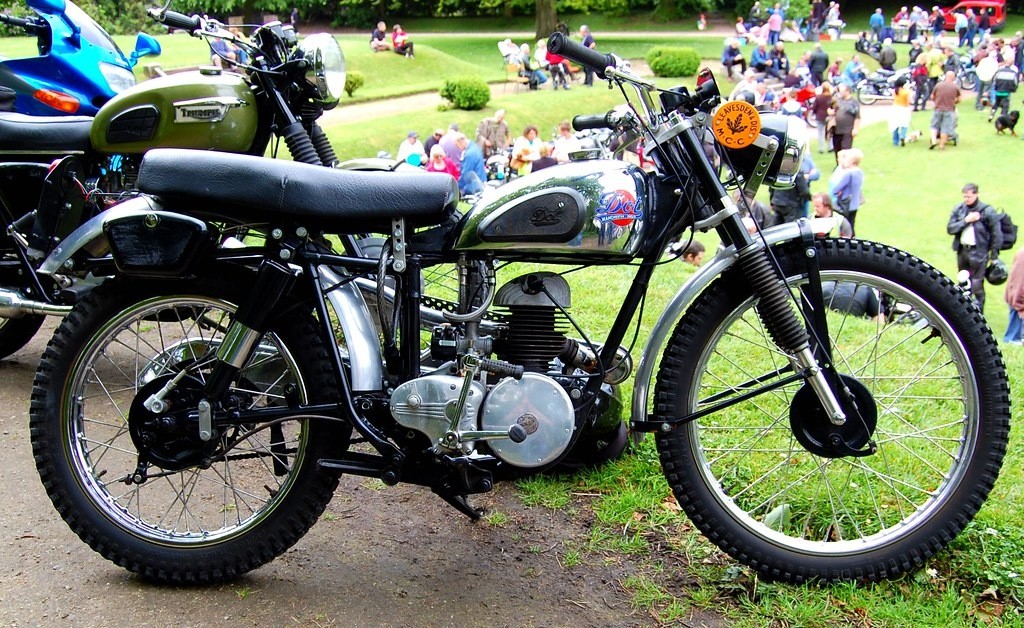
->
[904,130,923,144]
[995,111,1020,137]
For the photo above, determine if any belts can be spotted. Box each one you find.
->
[960,244,976,250]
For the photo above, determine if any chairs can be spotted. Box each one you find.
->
[503,59,579,94]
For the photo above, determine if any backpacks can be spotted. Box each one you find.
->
[977,204,1018,250]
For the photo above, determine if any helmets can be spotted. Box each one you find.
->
[985,259,1008,284]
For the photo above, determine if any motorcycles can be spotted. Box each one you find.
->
[25,22,1016,589]
[857,68,929,105]
[0,0,162,124]
[0,7,361,366]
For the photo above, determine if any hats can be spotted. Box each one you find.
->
[409,132,418,138]
[745,70,754,78]
[378,151,391,158]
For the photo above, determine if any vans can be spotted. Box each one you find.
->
[929,0,1007,34]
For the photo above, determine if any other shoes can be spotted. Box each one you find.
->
[901,138,904,146]
[930,145,936,149]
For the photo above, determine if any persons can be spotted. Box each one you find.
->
[505,24,596,90]
[892,75,912,147]
[568,214,613,246]
[392,24,414,59]
[947,183,1002,315]
[715,148,884,324]
[680,240,705,265]
[736,17,749,44]
[857,6,1024,122]
[732,42,866,169]
[1003,247,1024,343]
[721,41,746,82]
[291,8,299,33]
[370,22,390,50]
[929,71,961,150]
[396,124,486,197]
[513,117,582,176]
[748,0,846,45]
[211,28,250,71]
[476,108,511,153]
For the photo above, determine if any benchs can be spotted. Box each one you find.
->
[891,18,934,43]
